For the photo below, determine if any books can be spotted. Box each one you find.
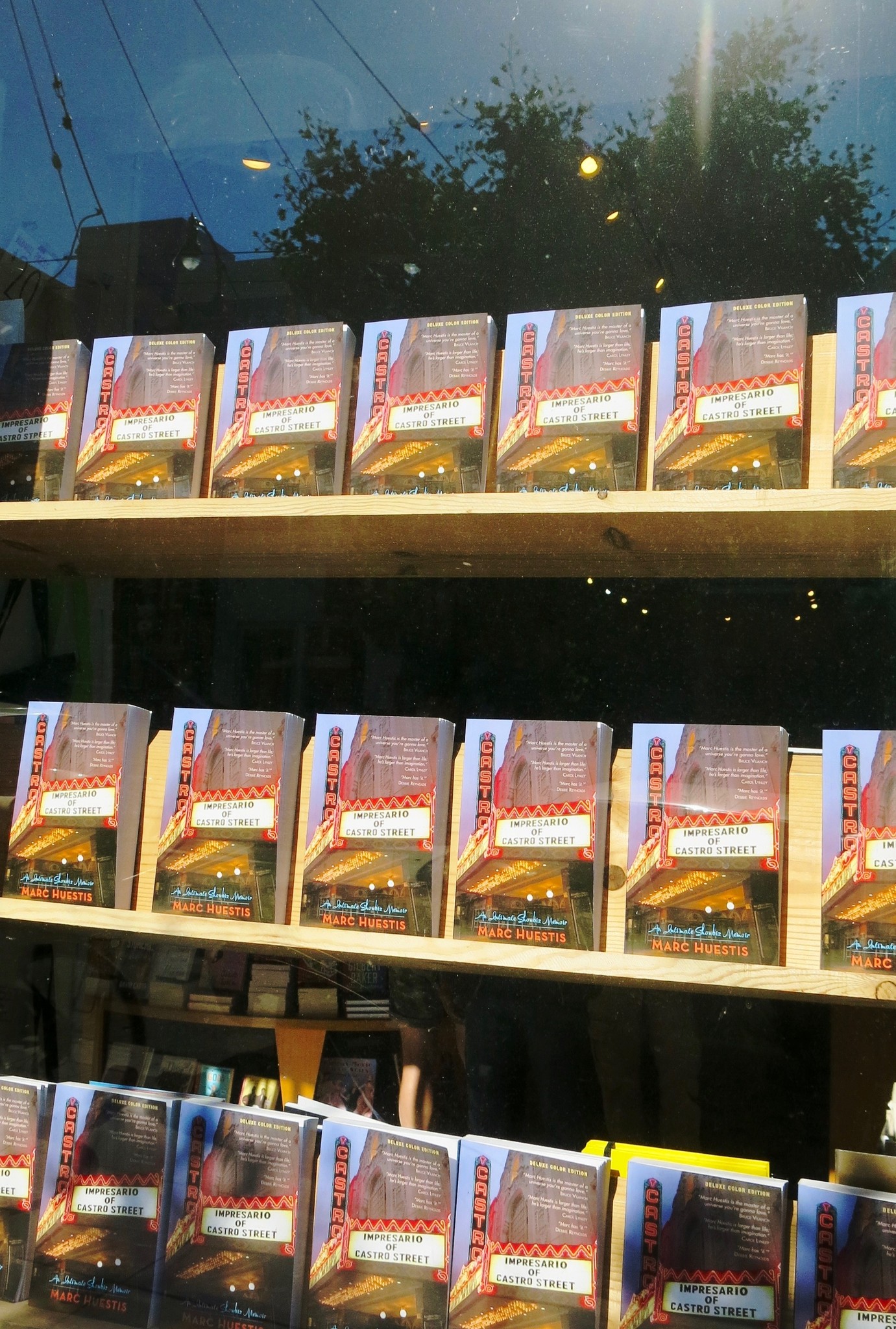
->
[74,333,216,500]
[1,701,153,910]
[199,1065,234,1103]
[624,722,790,966]
[284,1096,462,1328]
[447,1134,612,1329]
[314,1057,378,1118]
[0,339,91,500]
[349,312,498,495]
[210,321,356,499]
[300,714,457,938]
[820,728,895,973]
[153,1097,319,1329]
[652,294,808,491]
[82,937,392,1021]
[795,1174,896,1328]
[101,1042,197,1095]
[239,1075,280,1110]
[151,706,305,923]
[621,1160,789,1328]
[0,1072,57,1304]
[495,303,646,492]
[832,291,896,489]
[28,1079,225,1329]
[452,719,614,952]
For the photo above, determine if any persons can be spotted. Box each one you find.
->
[254,1088,267,1108]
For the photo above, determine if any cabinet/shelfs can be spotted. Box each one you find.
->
[0,486,896,1004]
